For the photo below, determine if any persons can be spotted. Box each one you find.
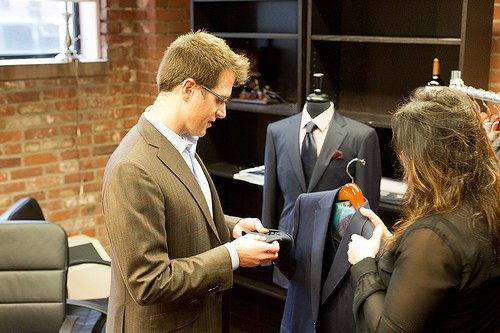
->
[100,29,280,332]
[347,86,500,333]
[262,102,382,289]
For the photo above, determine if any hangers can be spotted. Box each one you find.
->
[337,158,366,212]
[465,86,500,132]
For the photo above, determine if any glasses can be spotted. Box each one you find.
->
[179,77,230,109]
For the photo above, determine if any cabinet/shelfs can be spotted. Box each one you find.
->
[190,0,495,333]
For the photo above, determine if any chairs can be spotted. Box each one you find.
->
[0,197,112,333]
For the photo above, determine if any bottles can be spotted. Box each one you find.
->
[426,57,445,88]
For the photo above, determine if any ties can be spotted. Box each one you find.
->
[300,121,318,187]
[184,147,200,201]
